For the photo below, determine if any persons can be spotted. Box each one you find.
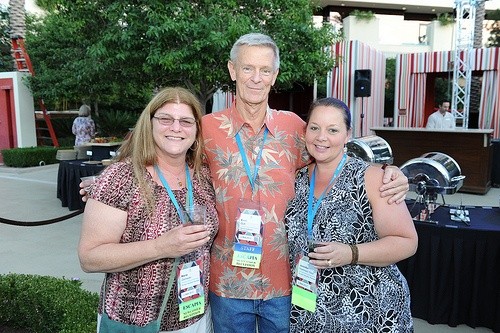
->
[385,79,394,88]
[426,99,455,129]
[79,33,409,333]
[285,97,418,333]
[78,86,220,333]
[72,105,95,150]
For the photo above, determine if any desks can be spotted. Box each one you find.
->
[57,158,115,211]
[393,198,500,333]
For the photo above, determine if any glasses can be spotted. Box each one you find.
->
[153,115,196,127]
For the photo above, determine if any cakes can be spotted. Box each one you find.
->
[93,138,109,143]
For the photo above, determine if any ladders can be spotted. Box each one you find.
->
[11,35,59,147]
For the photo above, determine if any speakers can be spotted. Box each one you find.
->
[354,69,372,97]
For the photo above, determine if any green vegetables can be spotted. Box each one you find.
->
[108,136,123,142]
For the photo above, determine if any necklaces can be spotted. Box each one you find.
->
[161,170,183,189]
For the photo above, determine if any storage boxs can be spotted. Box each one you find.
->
[92,141,122,161]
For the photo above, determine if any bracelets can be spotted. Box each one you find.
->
[382,164,388,170]
[349,245,358,266]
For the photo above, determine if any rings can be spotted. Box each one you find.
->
[326,260,331,267]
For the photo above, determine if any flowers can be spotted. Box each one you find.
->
[91,137,117,143]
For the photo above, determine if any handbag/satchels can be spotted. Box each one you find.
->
[99,313,160,333]
